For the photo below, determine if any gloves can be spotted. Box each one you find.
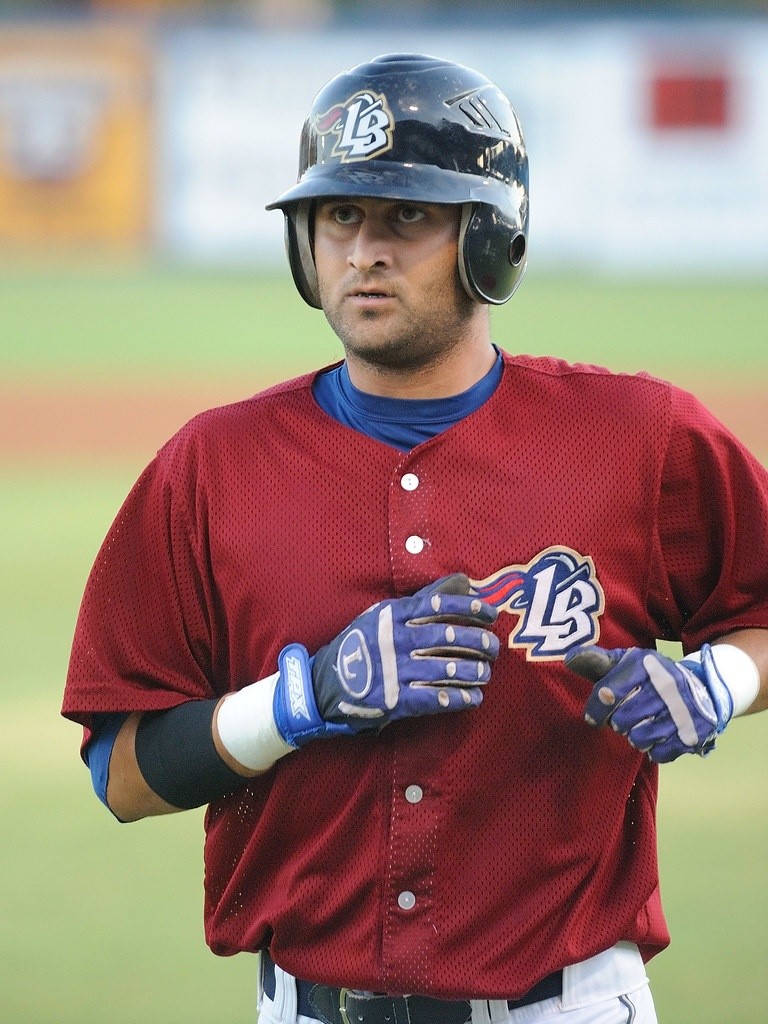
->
[272,574,499,748]
[565,644,734,763]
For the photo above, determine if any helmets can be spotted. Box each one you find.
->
[265,52,530,310]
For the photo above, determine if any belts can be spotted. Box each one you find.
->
[260,949,563,1024]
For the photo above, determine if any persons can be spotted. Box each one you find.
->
[61,54,767,1023]
